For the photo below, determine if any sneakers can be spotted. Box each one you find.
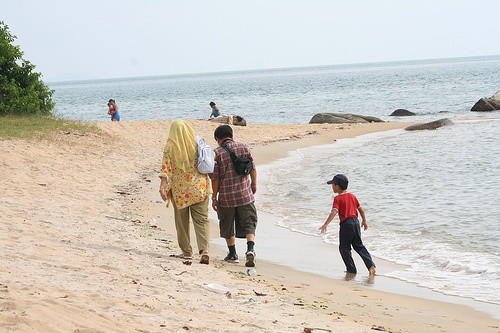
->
[224,253,239,263]
[246,250,256,267]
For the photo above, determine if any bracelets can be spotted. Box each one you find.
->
[212,198,216,200]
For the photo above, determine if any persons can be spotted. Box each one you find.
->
[107,99,120,121]
[319,174,376,275]
[210,125,258,267]
[210,102,220,117]
[159,119,210,263]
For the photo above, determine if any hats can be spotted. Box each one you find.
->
[326,174,348,189]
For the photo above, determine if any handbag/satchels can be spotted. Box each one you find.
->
[195,136,215,173]
[222,144,252,174]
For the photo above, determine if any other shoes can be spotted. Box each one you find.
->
[200,251,209,264]
[184,256,192,264]
